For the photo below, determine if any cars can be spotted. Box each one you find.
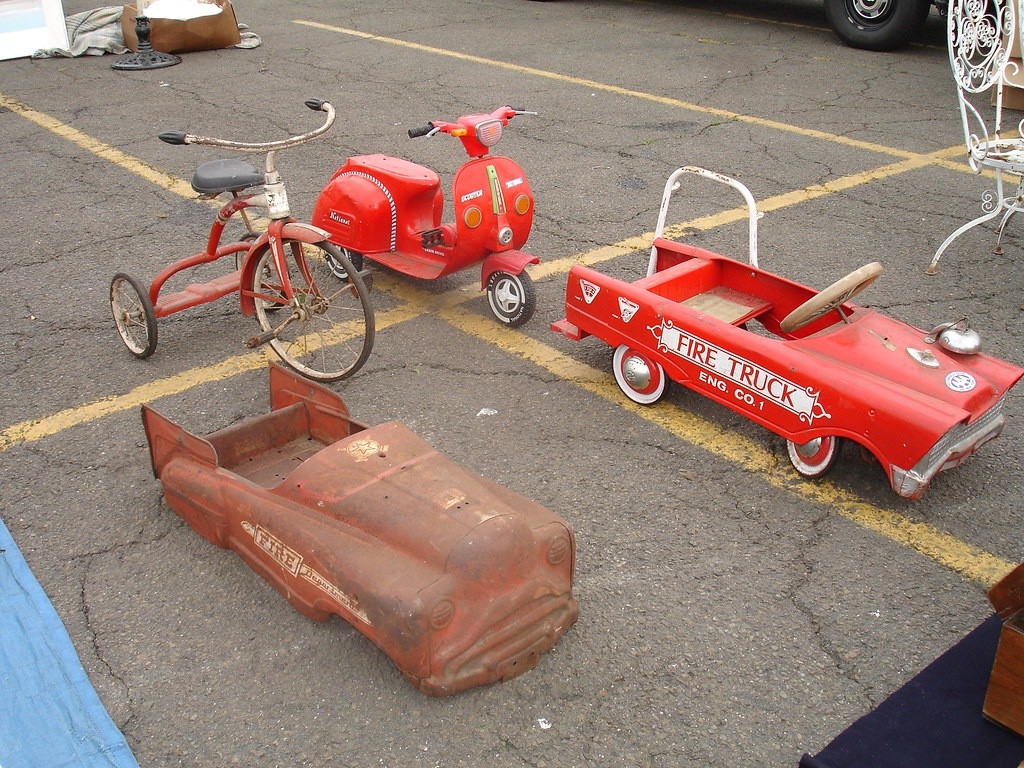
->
[551,164,1024,501]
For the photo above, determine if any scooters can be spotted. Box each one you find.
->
[311,104,539,327]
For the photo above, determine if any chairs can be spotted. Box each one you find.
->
[923,0,1024,276]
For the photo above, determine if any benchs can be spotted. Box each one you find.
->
[678,284,773,330]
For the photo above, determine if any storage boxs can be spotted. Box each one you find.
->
[122,0,241,54]
[982,561,1024,737]
[1002,1,1023,59]
[990,57,1024,110]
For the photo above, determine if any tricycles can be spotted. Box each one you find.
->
[110,98,378,386]
